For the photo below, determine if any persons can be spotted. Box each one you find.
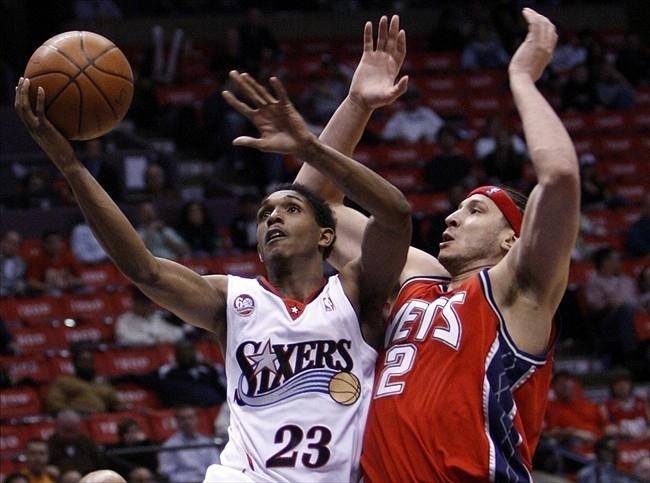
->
[429,185,649,483]
[291,8,580,483]
[1,0,272,483]
[291,53,355,123]
[14,71,410,482]
[378,0,648,213]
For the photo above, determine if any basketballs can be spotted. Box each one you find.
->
[25,31,134,141]
